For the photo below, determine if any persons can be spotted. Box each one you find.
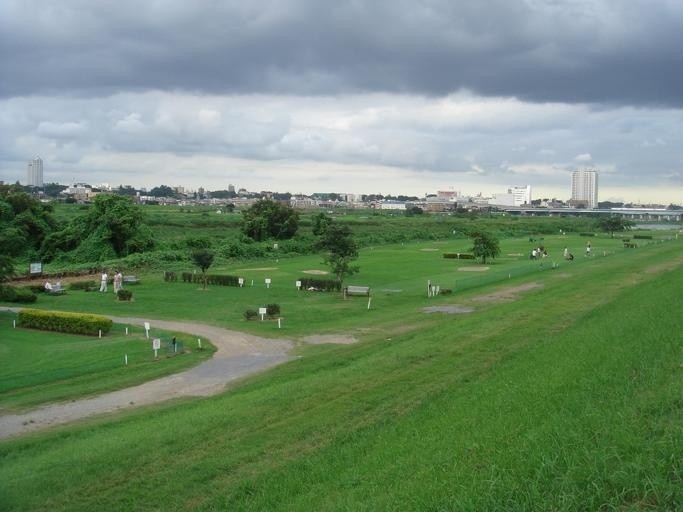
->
[531,243,549,261]
[113,270,121,294]
[584,240,592,257]
[44,279,52,290]
[563,245,567,257]
[118,271,123,289]
[99,270,108,292]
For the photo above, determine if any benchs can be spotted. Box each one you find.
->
[343,285,370,296]
[122,274,137,285]
[48,284,65,295]
